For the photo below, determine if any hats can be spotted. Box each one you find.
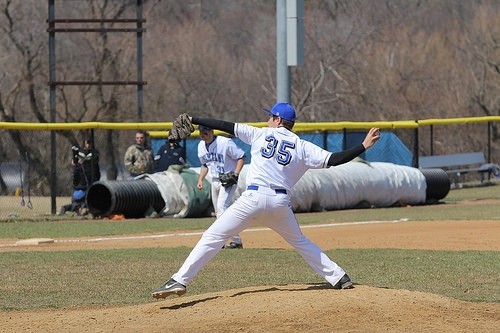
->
[262,102,296,122]
[197,125,212,131]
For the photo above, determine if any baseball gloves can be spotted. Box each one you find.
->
[168,112,194,142]
[220,170,238,189]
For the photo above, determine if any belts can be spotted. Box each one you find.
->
[247,185,287,194]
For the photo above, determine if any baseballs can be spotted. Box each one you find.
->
[374,129,380,136]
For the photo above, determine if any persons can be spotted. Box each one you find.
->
[152,137,186,173]
[152,102,380,299]
[196,125,247,249]
[123,131,154,178]
[71,137,101,183]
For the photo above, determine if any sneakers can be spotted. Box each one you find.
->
[339,274,353,290]
[150,278,187,301]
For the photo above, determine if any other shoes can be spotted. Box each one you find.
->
[225,242,243,250]
